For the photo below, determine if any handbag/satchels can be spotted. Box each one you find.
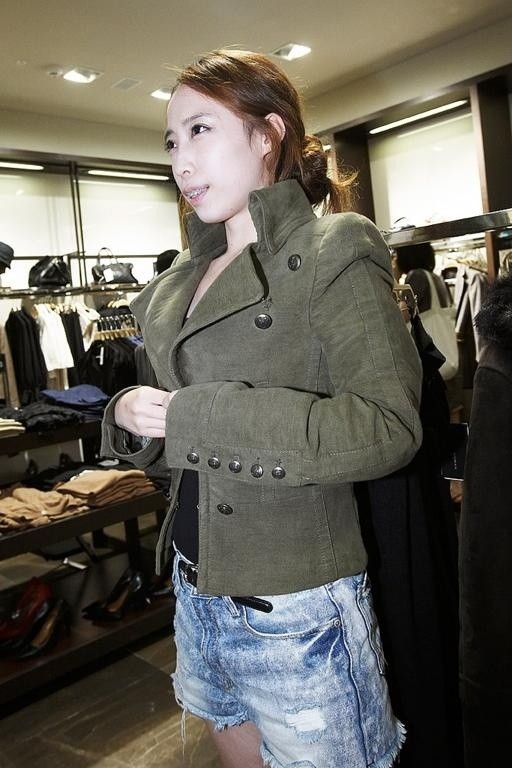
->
[404,269,462,381]
[28,255,71,287]
[91,261,138,284]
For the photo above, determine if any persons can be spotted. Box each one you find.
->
[100,49,422,767]
[389,224,463,423]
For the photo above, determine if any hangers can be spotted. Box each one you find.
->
[438,242,507,282]
[85,289,142,342]
[3,292,83,317]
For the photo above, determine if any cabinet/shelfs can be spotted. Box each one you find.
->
[1,409,178,719]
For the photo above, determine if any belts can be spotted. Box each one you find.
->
[176,559,273,612]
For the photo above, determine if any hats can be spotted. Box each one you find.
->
[0,242,14,269]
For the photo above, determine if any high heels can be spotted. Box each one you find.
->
[1,599,56,659]
[81,566,152,622]
[18,598,74,662]
[0,575,54,645]
